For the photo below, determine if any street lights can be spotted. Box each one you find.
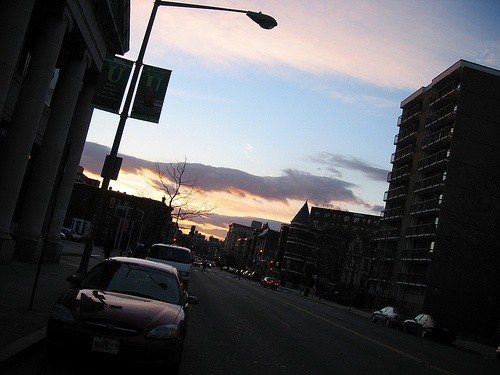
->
[67,0,278,288]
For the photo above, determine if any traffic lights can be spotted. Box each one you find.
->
[259,247,263,254]
[171,237,176,244]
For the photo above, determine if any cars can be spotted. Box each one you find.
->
[60,233,66,240]
[260,277,279,290]
[193,255,216,268]
[222,265,262,282]
[45,254,199,375]
[371,305,405,328]
[403,313,458,344]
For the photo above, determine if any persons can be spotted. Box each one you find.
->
[220,262,224,271]
[102,237,114,259]
[201,262,207,272]
[233,269,240,280]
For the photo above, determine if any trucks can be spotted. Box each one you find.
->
[145,243,195,291]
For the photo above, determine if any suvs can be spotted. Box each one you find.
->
[62,227,75,240]
